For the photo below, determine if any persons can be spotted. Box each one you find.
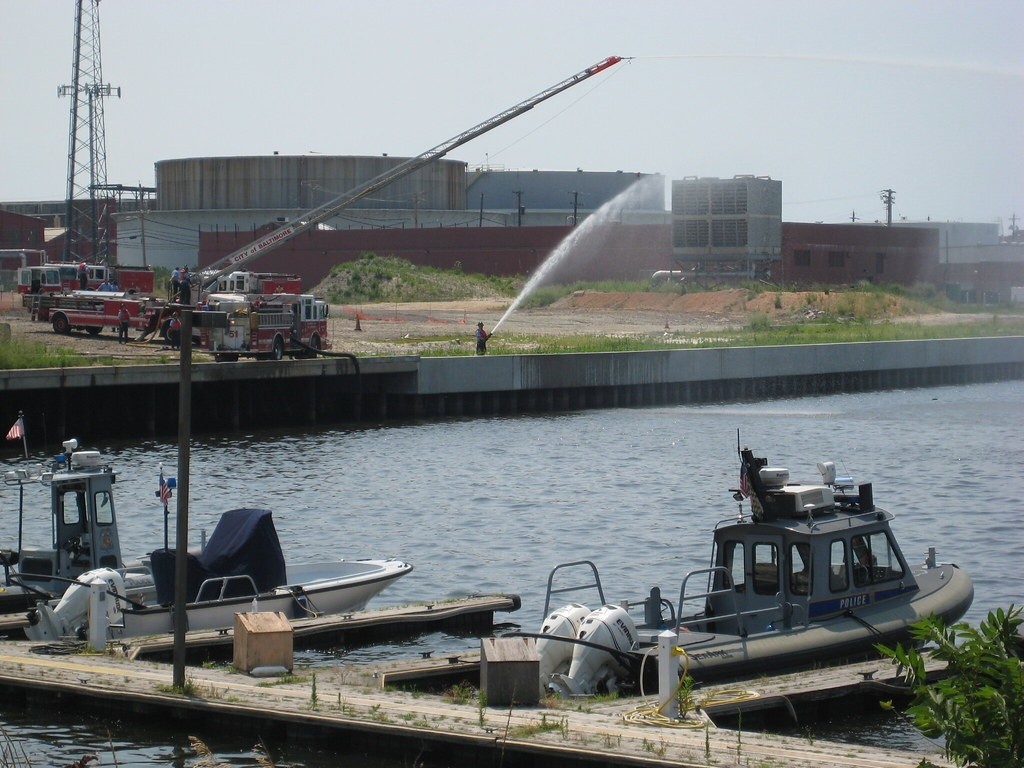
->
[291,296,301,321]
[77,259,90,290]
[253,296,260,313]
[117,303,130,344]
[168,312,181,349]
[97,278,119,292]
[169,264,194,305]
[475,322,491,355]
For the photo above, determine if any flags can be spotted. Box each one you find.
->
[739,464,764,519]
[159,472,172,507]
[5,417,24,440]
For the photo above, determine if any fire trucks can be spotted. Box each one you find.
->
[15,259,165,335]
[161,53,632,367]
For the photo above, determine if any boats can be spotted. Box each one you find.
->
[495,426,977,716]
[6,505,414,649]
[0,436,208,611]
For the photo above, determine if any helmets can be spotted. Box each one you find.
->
[121,303,125,307]
[180,269,185,273]
[173,312,179,315]
[477,322,484,326]
